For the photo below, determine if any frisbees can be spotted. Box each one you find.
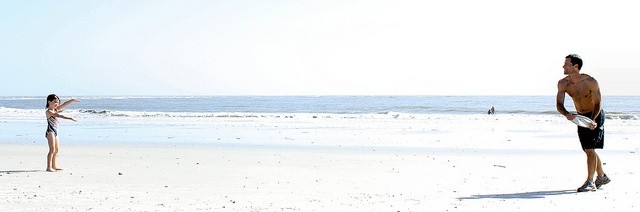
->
[569,114,594,129]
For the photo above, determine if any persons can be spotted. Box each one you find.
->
[44,94,80,172]
[556,54,611,192]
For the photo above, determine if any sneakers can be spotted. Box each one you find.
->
[578,179,596,190]
[594,173,610,189]
